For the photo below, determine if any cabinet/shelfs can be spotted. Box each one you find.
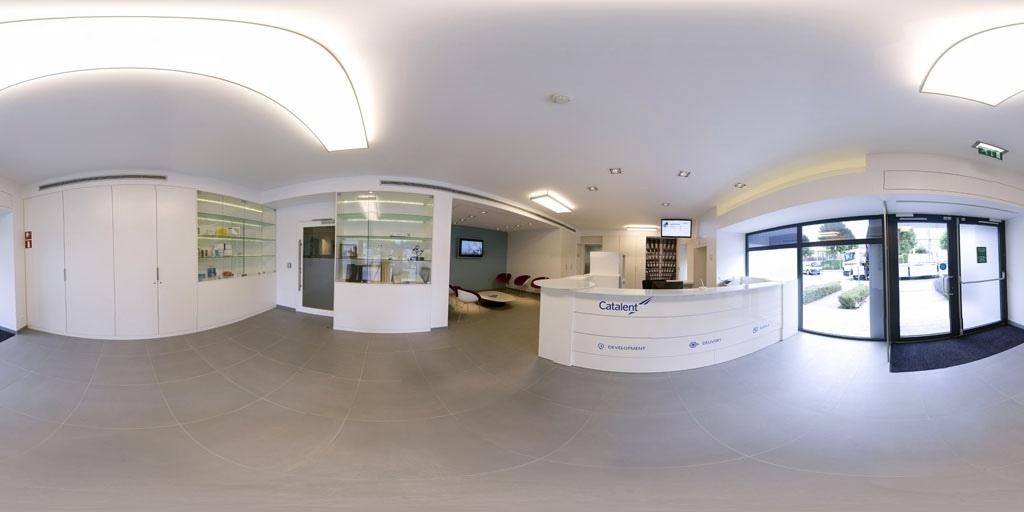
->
[645,236,678,281]
[196,189,277,283]
[21,184,197,342]
[335,189,435,285]
[619,234,645,289]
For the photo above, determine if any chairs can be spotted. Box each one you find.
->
[449,284,482,321]
[496,272,550,298]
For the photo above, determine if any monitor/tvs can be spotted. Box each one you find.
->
[459,238,484,257]
[652,280,683,288]
[661,219,692,238]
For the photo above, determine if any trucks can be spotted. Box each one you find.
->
[842,248,869,280]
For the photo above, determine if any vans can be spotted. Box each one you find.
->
[802,256,822,275]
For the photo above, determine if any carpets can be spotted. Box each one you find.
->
[888,324,1024,373]
